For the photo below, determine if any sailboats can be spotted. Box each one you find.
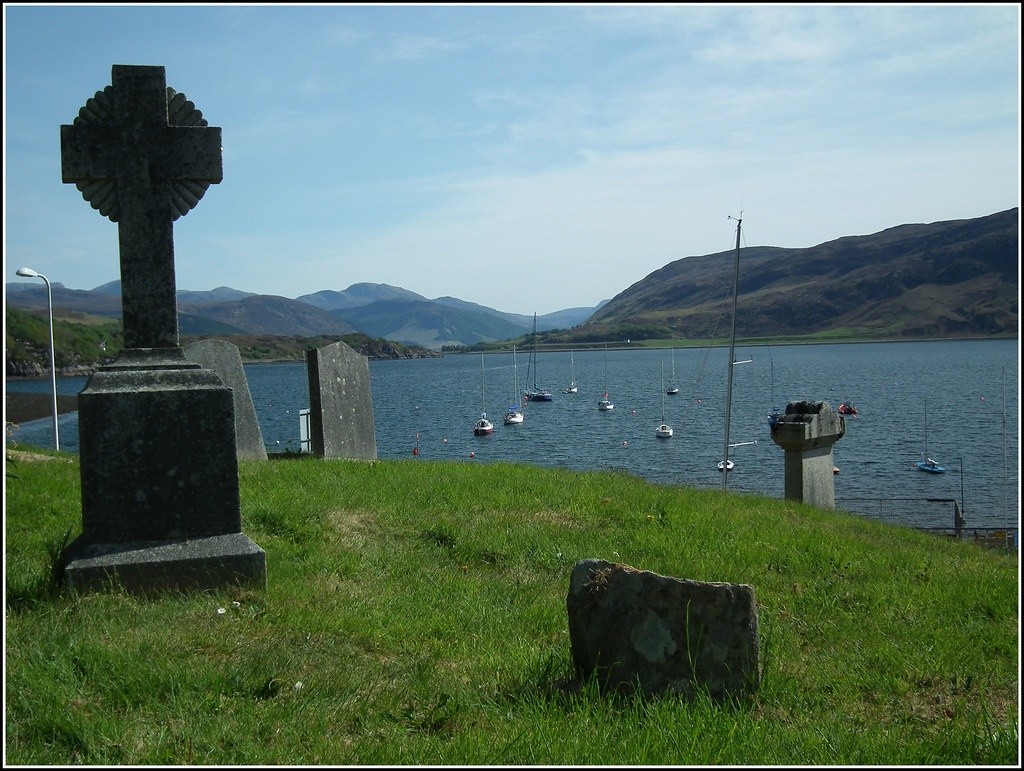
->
[566,349,578,393]
[503,345,524,425]
[915,395,946,473]
[598,345,614,410]
[522,312,553,401]
[655,361,673,438]
[474,351,493,435]
[667,346,679,395]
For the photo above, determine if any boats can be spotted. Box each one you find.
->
[838,402,857,415]
[718,460,734,471]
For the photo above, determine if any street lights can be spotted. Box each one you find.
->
[16,267,60,451]
